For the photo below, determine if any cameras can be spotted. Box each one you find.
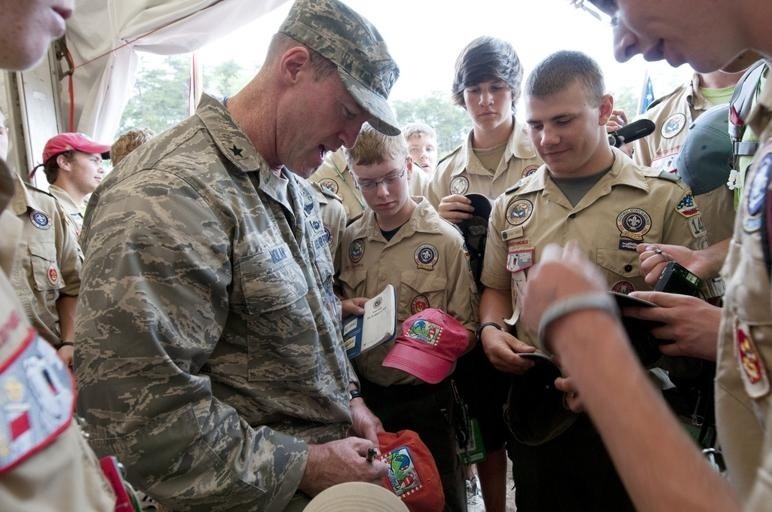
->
[608,119,655,149]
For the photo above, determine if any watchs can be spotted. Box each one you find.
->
[476,322,504,341]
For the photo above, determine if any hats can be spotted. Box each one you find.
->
[42,133,113,164]
[502,350,583,447]
[302,482,413,512]
[370,429,447,512]
[280,1,403,137]
[678,104,734,196]
[381,308,473,382]
[457,194,493,291]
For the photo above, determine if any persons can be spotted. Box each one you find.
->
[35,133,113,247]
[286,173,347,274]
[0,161,88,371]
[523,0,771,512]
[0,0,162,512]
[481,53,724,512]
[426,39,545,512]
[73,0,401,512]
[109,126,156,172]
[404,121,439,183]
[607,55,763,195]
[337,125,479,512]
[313,136,373,227]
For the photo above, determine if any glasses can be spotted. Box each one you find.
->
[572,0,602,22]
[355,164,409,192]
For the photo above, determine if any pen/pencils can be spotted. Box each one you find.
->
[366,447,377,466]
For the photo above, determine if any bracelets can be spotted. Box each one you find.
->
[535,291,616,355]
